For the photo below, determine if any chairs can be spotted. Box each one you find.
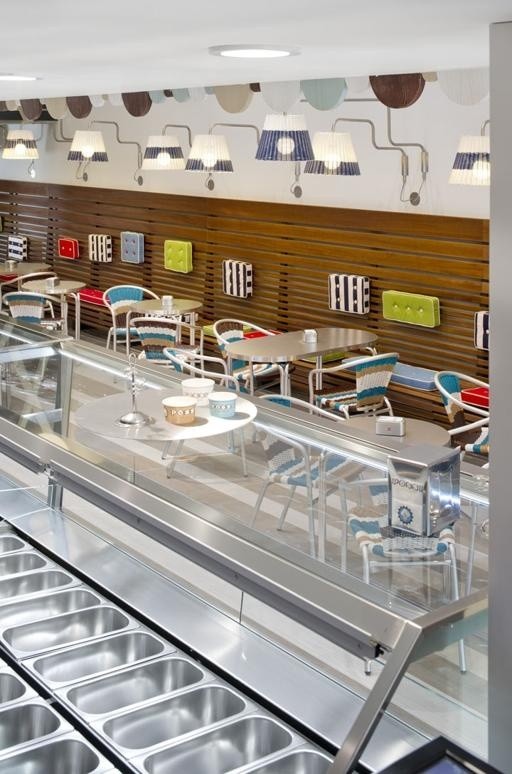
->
[0,255,492,676]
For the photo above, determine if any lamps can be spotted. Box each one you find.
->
[205,40,302,69]
[0,111,490,207]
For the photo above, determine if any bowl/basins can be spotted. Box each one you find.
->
[161,394,198,425]
[206,391,237,418]
[180,378,214,404]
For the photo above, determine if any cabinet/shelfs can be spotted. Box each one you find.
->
[1,312,493,773]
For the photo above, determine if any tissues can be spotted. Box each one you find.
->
[162,294,174,306]
[5,259,17,269]
[375,415,407,437]
[302,329,318,343]
[46,276,60,288]
